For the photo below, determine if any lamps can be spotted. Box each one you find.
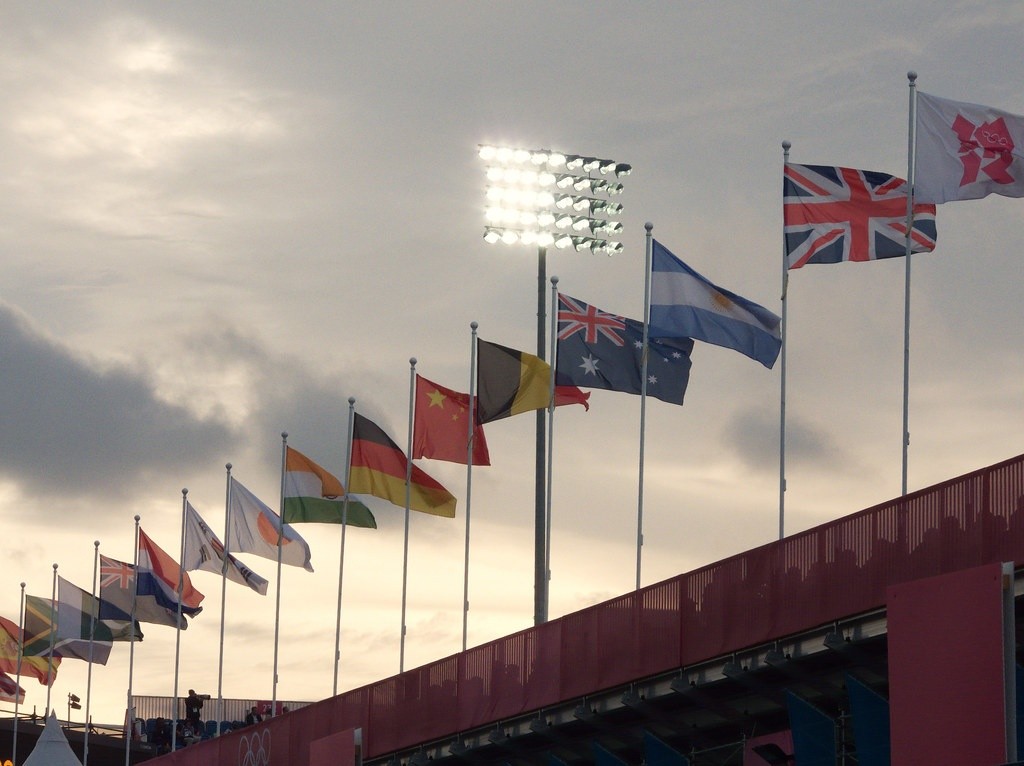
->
[721,654,743,680]
[408,751,432,766]
[823,623,846,652]
[763,641,783,669]
[448,741,471,757]
[619,690,643,707]
[529,715,551,734]
[671,670,694,694]
[573,704,592,719]
[751,742,794,766]
[487,728,511,746]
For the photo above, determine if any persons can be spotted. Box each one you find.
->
[184,689,204,733]
[246,705,289,726]
[153,717,202,755]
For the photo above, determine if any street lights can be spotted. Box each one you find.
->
[479,143,634,626]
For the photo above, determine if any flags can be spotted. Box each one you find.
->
[226,478,315,574]
[412,374,491,467]
[137,528,206,619]
[23,594,113,665]
[347,412,457,519]
[183,501,268,596]
[97,557,188,631]
[56,576,144,642]
[783,163,938,269]
[282,445,378,529]
[0,616,62,688]
[476,338,591,427]
[554,293,695,406]
[647,239,782,370]
[914,91,1024,205]
[0,667,27,705]
